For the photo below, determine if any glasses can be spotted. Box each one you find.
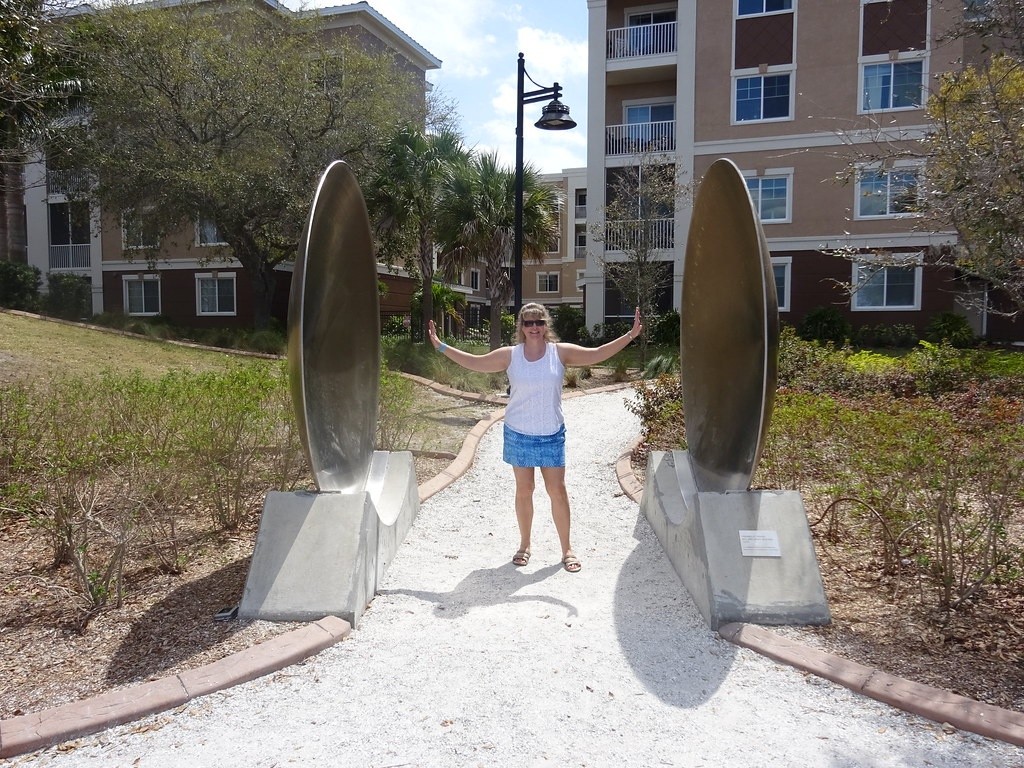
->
[523,320,545,327]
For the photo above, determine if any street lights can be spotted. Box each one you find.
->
[514,82,577,321]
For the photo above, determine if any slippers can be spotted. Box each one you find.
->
[562,555,581,572]
[512,549,531,566]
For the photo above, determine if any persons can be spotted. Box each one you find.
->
[428,302,643,573]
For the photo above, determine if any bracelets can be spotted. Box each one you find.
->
[439,342,447,352]
[628,331,633,340]
